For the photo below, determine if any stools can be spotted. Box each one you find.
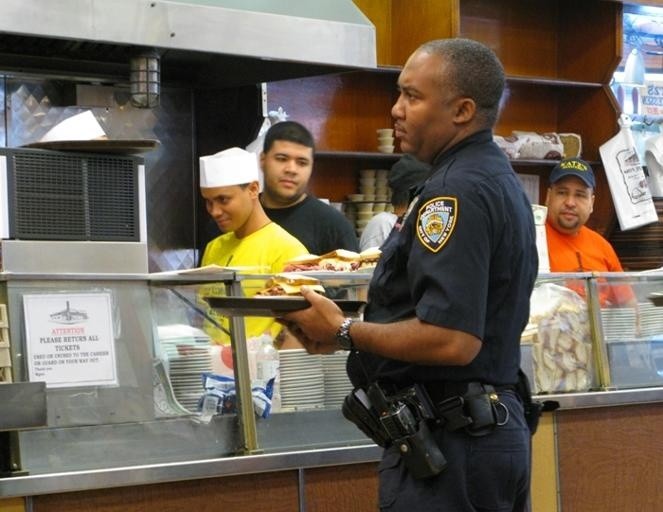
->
[438,384,520,412]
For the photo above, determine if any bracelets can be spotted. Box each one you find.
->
[375,128,394,154]
[348,165,395,239]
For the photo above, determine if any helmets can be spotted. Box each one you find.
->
[160,344,356,413]
[597,306,662,343]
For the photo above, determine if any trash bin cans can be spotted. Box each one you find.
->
[337,317,351,351]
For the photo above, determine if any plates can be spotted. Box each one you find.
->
[268,0,627,245]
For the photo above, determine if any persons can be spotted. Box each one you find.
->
[273,36,533,509]
[195,147,309,352]
[258,120,360,253]
[360,155,431,257]
[543,157,640,309]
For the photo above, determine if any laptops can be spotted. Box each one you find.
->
[273,249,381,293]
[520,304,589,392]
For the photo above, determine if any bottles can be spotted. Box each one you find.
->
[40,110,107,142]
[383,155,430,193]
[199,145,260,188]
[548,156,596,188]
